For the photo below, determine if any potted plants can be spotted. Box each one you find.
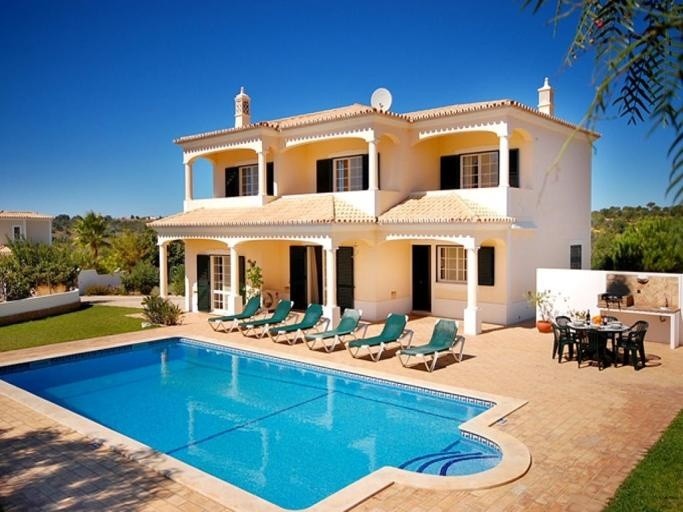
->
[526,287,568,333]
[140,293,183,326]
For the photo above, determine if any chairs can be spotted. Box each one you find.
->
[549,314,650,371]
[208,296,466,373]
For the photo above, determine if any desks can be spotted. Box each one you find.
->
[596,305,680,349]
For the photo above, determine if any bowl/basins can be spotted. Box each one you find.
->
[610,322,622,329]
[574,320,584,326]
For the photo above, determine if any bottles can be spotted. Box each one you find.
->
[585,310,590,325]
[602,310,607,325]
